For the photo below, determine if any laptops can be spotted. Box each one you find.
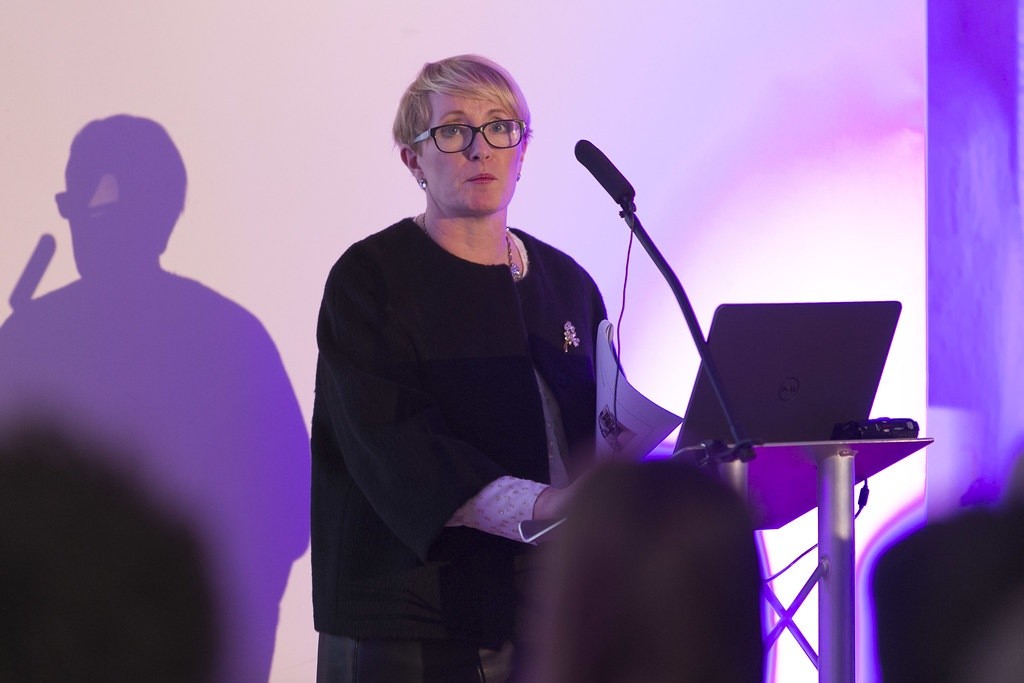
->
[674,300,903,458]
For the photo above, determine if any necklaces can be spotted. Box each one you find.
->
[423,214,521,279]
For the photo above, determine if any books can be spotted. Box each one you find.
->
[596,319,682,464]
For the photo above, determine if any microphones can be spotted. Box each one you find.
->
[574,139,636,211]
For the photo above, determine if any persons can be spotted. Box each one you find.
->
[0,427,214,683]
[870,510,1024,683]
[544,463,764,683]
[311,54,629,683]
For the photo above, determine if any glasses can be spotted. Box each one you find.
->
[409,117,529,155]
